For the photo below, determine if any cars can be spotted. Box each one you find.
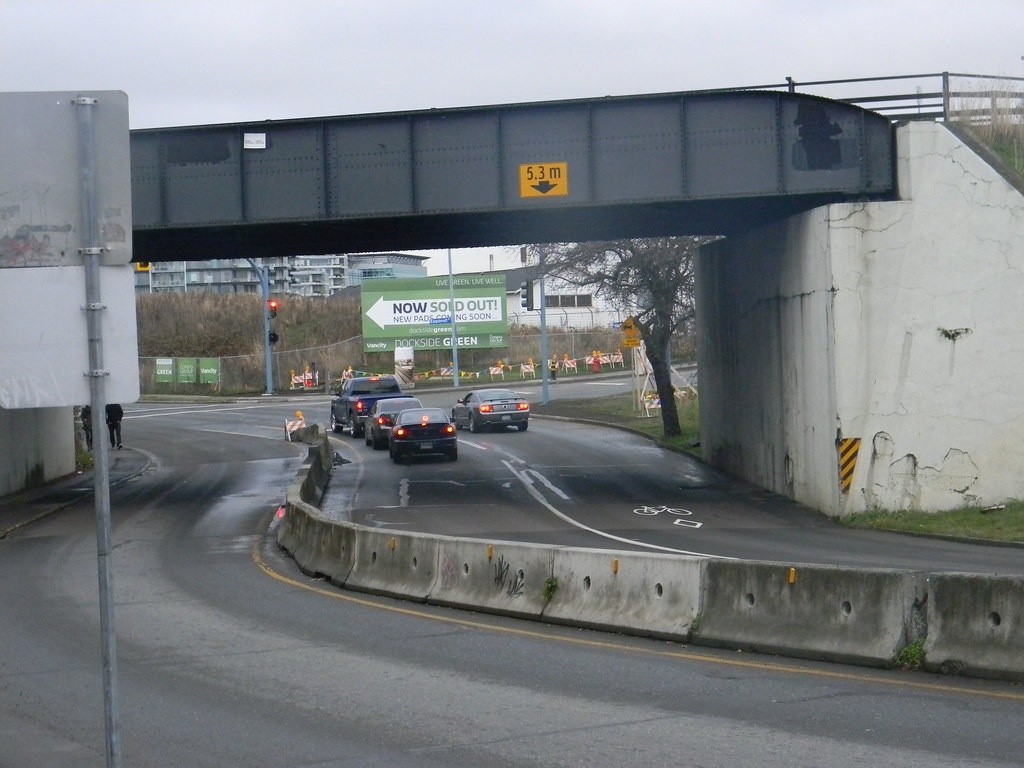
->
[361,396,425,451]
[452,388,530,433]
[383,408,459,464]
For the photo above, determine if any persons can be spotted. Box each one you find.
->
[105,404,123,450]
[81,405,93,451]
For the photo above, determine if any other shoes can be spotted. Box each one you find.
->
[112,445,115,448]
[117,444,122,449]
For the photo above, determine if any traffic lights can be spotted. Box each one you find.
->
[270,301,276,317]
[520,279,534,310]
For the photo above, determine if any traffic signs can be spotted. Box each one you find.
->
[359,271,510,353]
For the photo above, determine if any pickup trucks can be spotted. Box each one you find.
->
[330,376,414,438]
